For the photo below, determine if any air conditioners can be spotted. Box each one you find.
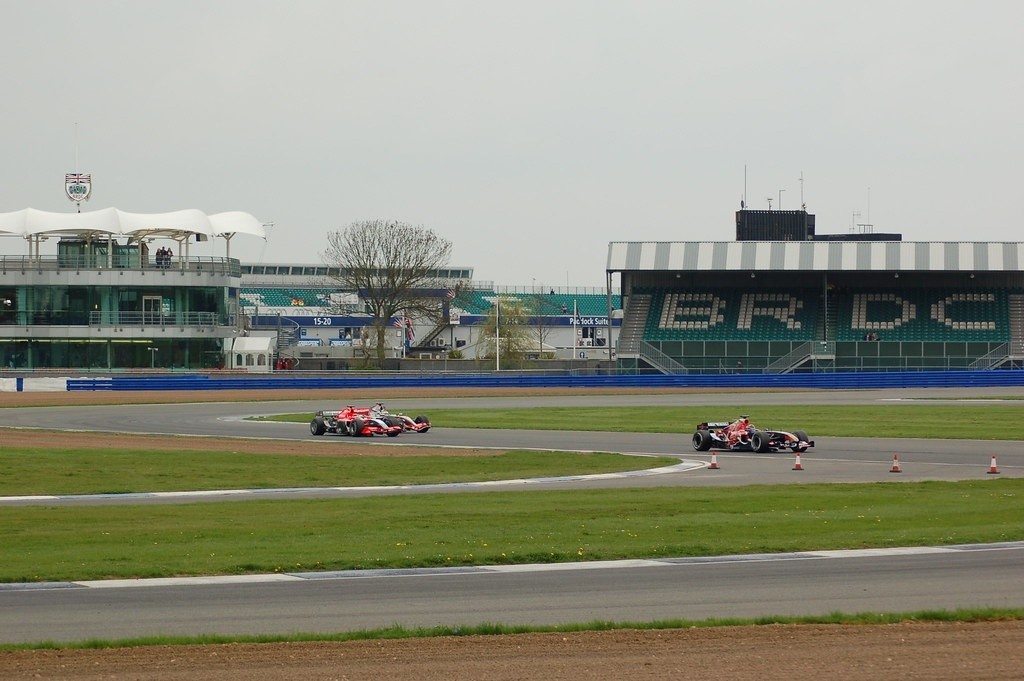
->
[419,353,432,360]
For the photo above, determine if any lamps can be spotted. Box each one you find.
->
[970,273,974,279]
[894,273,899,278]
[751,273,755,278]
[675,273,682,278]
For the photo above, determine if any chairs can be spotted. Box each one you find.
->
[837,286,1024,342]
[633,287,819,341]
[449,291,608,321]
[611,294,622,309]
[162,288,337,316]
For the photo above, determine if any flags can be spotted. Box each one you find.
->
[404,310,415,346]
[445,287,456,302]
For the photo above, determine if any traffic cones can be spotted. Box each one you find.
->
[792,454,805,470]
[708,452,721,469]
[987,456,1001,474]
[889,454,903,473]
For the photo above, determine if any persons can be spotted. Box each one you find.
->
[156,248,162,268]
[166,248,173,269]
[160,246,167,268]
